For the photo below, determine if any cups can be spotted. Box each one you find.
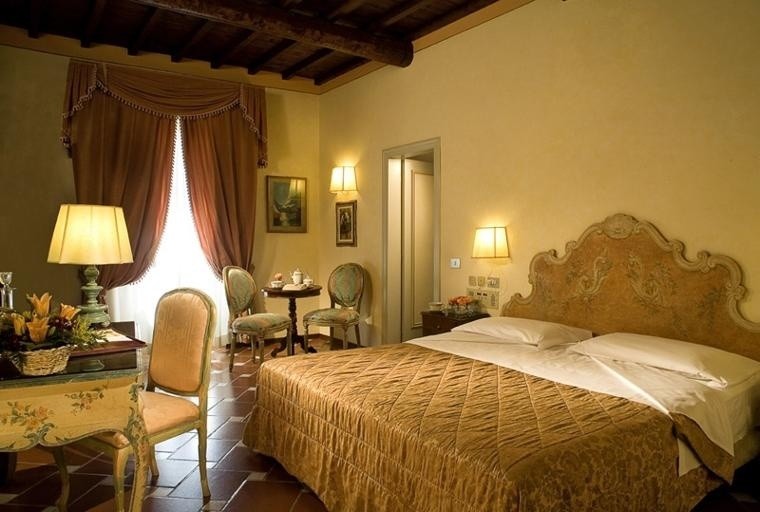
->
[303,279,313,288]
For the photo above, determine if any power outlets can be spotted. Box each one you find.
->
[487,277,499,288]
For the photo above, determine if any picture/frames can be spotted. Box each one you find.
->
[265,173,308,234]
[335,200,357,247]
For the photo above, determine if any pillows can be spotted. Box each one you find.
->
[452,314,595,349]
[567,333,759,388]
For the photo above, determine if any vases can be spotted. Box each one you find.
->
[459,304,466,313]
[450,304,459,312]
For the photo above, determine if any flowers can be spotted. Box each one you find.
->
[457,297,473,304]
[6,291,111,347]
[448,296,462,304]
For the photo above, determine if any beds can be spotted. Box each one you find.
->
[244,212,760,512]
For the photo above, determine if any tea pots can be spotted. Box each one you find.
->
[289,266,302,285]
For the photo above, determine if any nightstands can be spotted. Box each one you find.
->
[420,311,492,343]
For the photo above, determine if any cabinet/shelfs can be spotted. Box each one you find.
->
[0,324,147,379]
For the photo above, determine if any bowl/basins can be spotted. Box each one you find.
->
[429,302,443,311]
[270,281,283,288]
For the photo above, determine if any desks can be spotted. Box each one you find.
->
[260,283,322,357]
[1,372,149,510]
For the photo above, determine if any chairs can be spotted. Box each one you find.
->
[221,264,293,372]
[47,287,218,511]
[302,263,366,353]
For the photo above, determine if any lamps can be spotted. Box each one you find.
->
[43,200,134,327]
[470,224,511,259]
[327,164,360,194]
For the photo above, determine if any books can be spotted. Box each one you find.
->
[281,283,314,291]
